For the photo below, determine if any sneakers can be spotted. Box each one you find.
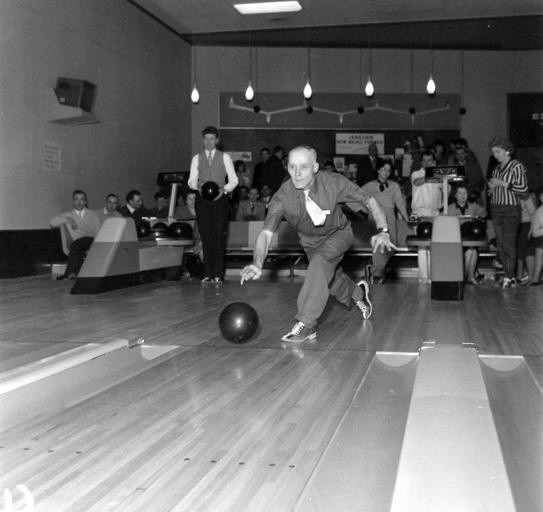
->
[281,321,317,343]
[202,276,222,283]
[355,280,373,320]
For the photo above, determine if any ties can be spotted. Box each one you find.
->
[303,189,327,226]
[208,151,212,165]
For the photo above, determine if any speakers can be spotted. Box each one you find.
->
[56,75,97,113]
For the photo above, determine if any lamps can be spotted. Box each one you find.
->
[244,25,253,101]
[189,32,200,103]
[363,21,374,97]
[302,25,312,99]
[426,21,436,95]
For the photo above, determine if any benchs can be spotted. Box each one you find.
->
[224,218,498,284]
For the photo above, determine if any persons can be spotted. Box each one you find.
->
[354,137,543,289]
[230,146,288,223]
[50,190,101,283]
[236,144,398,341]
[187,125,239,285]
[98,191,196,230]
